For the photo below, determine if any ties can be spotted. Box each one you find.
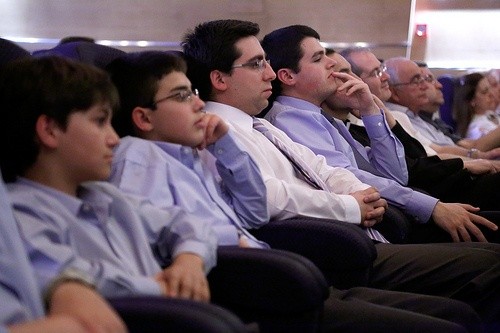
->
[252,115,390,244]
[321,109,390,179]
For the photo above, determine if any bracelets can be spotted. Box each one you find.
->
[467,148,479,158]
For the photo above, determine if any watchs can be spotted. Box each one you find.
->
[42,267,99,309]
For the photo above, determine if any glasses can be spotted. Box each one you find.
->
[151,88,199,109]
[209,60,271,73]
[389,76,425,87]
[361,64,387,79]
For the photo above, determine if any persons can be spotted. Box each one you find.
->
[0,20,500,333]
